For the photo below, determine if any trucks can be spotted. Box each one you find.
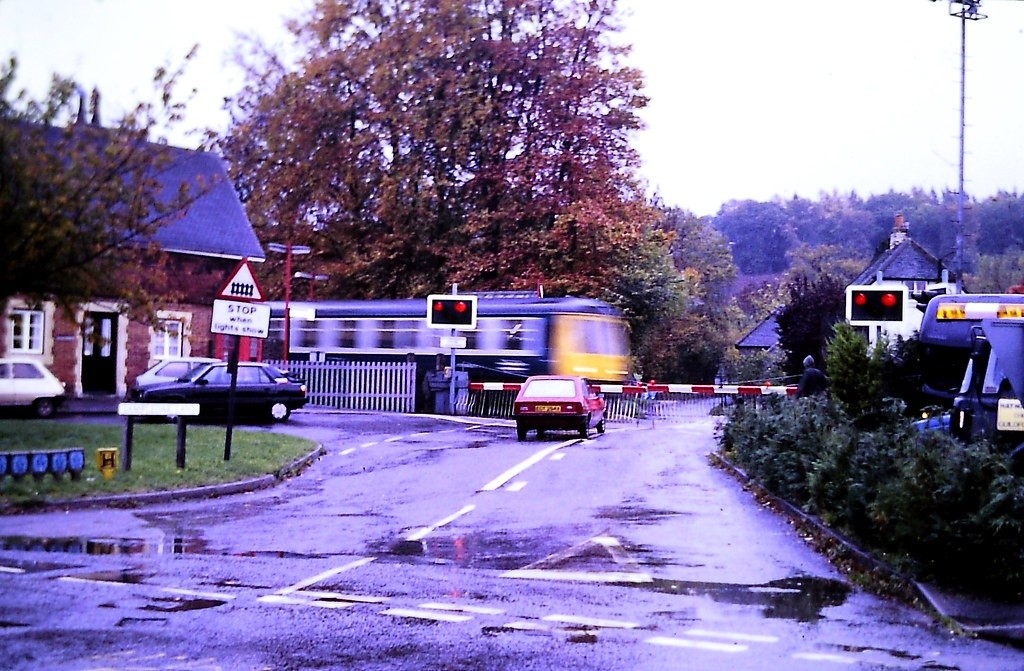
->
[903,292,1024,472]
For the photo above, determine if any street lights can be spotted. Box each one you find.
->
[294,271,330,302]
[269,239,312,370]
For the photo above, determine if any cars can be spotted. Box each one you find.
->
[136,357,225,390]
[513,375,608,439]
[0,356,69,421]
[129,360,311,424]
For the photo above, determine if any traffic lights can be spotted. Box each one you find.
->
[911,286,942,311]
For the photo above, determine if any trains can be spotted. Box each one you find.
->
[248,290,631,394]
